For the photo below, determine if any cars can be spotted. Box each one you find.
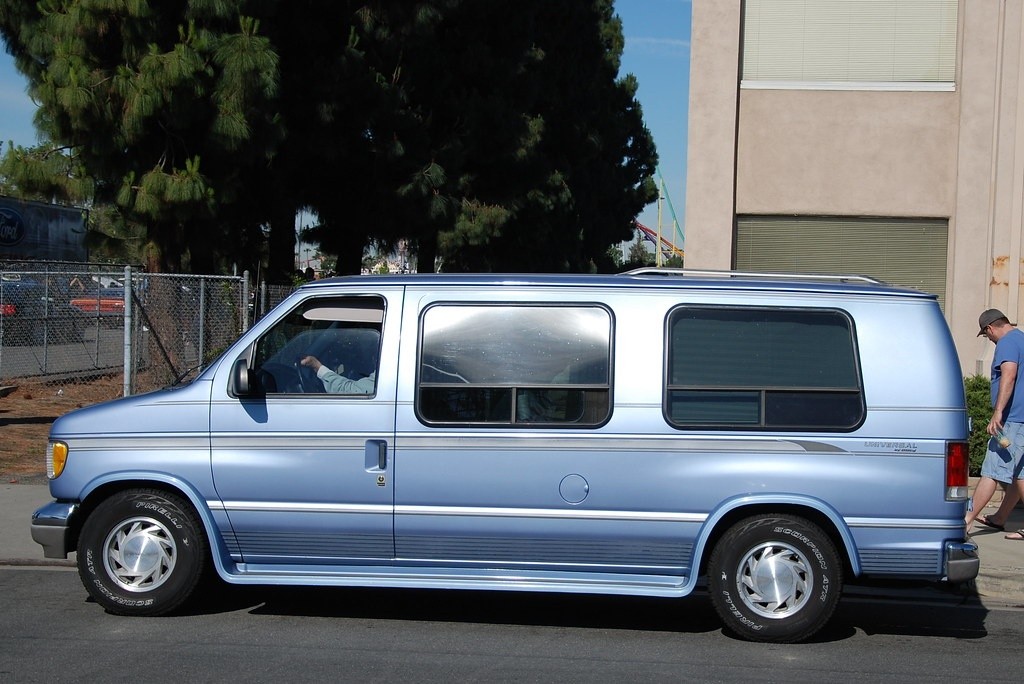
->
[0,274,256,345]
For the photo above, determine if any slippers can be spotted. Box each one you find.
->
[1005,529,1024,540]
[974,514,1006,531]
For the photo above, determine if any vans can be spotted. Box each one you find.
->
[28,267,983,645]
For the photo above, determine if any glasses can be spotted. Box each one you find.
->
[983,327,988,337]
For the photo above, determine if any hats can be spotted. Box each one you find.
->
[977,309,1006,337]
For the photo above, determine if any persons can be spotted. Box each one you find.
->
[966,309,1024,540]
[295,355,375,395]
[68,275,85,298]
[306,268,316,281]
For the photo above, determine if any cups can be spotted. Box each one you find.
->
[993,430,1011,449]
